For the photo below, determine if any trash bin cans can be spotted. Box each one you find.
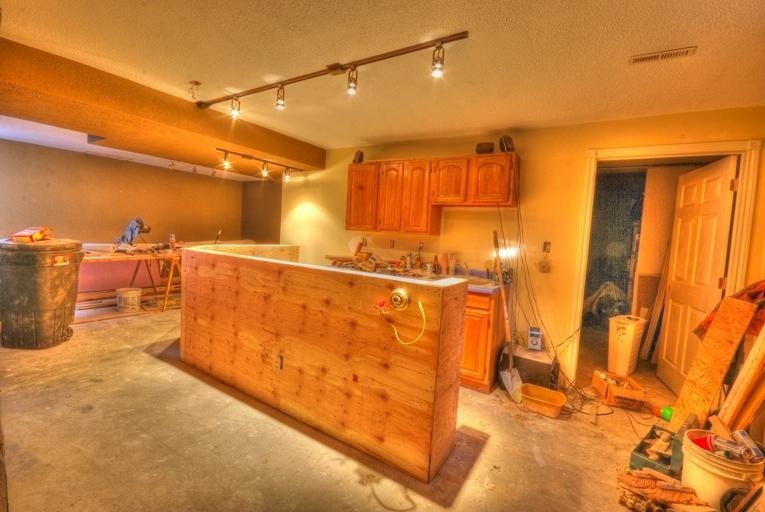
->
[607,315,648,377]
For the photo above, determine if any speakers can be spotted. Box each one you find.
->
[352,150,363,163]
[499,135,514,152]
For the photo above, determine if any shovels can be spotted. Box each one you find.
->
[492,231,526,405]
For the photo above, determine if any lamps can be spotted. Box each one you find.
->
[262,162,270,177]
[277,88,285,109]
[231,99,241,118]
[347,68,358,94]
[285,168,291,180]
[224,152,231,169]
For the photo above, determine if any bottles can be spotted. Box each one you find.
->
[449,254,455,275]
[407,252,412,267]
[733,430,764,463]
[435,256,439,273]
[442,252,448,274]
[714,436,752,459]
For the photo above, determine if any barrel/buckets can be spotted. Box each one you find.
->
[115,287,141,313]
[681,429,765,508]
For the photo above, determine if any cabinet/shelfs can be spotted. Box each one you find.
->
[431,152,521,208]
[460,270,509,394]
[378,160,441,237]
[346,163,377,231]
